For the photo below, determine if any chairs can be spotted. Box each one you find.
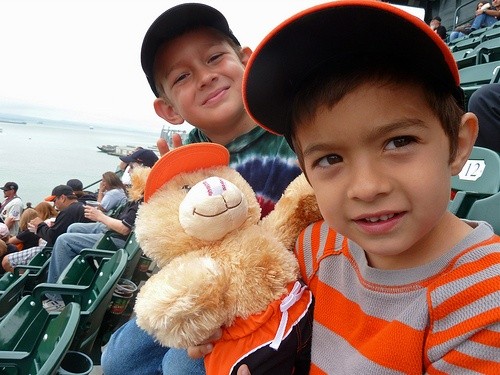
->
[0,232,143,375]
[449,20,500,234]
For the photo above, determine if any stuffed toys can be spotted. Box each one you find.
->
[127,142,323,375]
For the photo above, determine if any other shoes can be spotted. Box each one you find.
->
[460,26,472,35]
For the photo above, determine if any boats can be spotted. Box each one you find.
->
[96,144,124,158]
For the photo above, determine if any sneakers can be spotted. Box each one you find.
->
[41,298,66,312]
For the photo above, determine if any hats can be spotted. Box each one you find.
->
[119,149,159,169]
[44,185,74,201]
[242,0,459,153]
[140,3,240,97]
[67,178,83,191]
[429,16,441,22]
[0,222,10,239]
[0,182,18,191]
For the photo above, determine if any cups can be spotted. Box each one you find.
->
[109,278,138,314]
[85,200,100,210]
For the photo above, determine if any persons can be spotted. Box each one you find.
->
[187,1,500,375]
[0,0,500,375]
